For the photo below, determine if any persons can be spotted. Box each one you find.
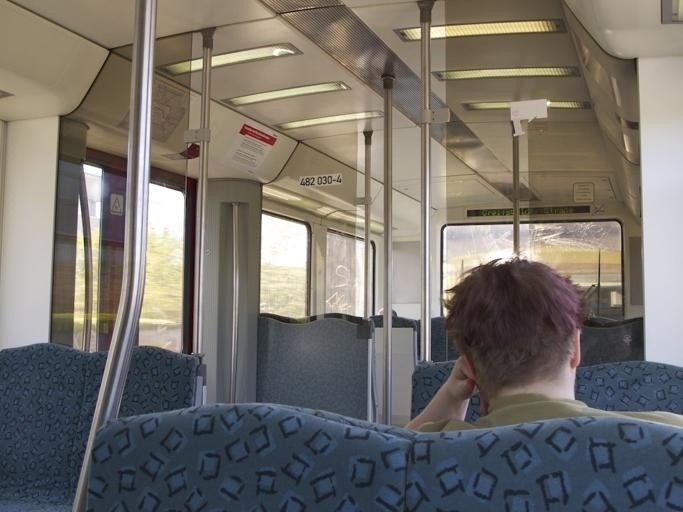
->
[402,258,683,428]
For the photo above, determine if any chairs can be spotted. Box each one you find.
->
[59,346,205,511]
[432,317,459,362]
[408,417,683,512]
[411,362,482,424]
[580,317,644,367]
[369,317,418,428]
[256,313,376,421]
[0,343,86,512]
[572,361,683,415]
[88,402,409,511]
